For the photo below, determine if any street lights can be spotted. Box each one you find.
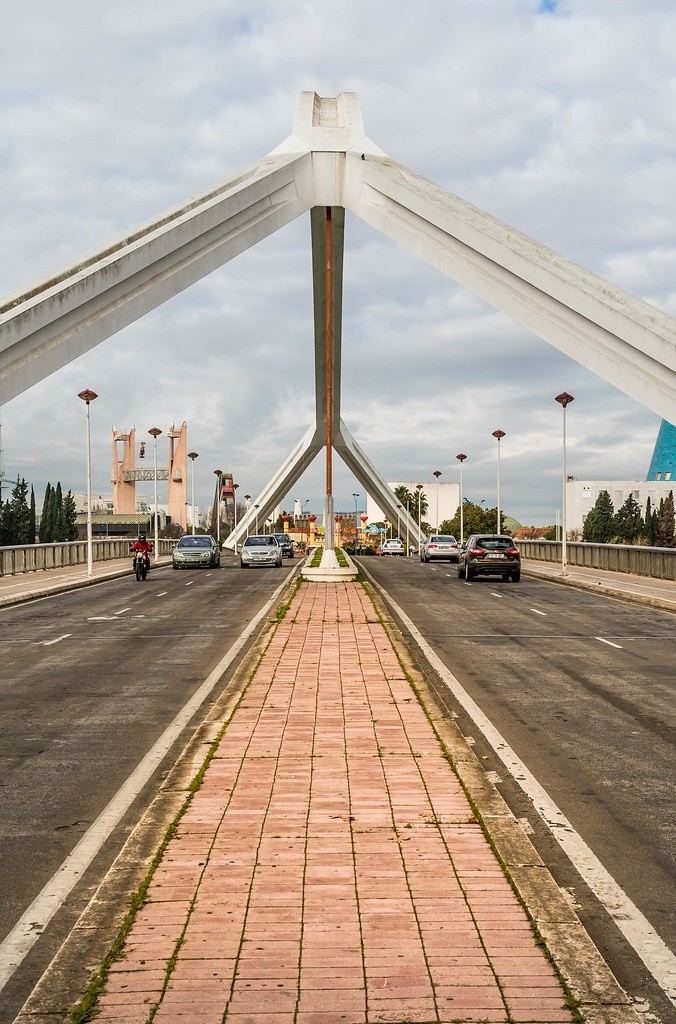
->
[268,519,272,534]
[384,520,388,541]
[491,428,507,536]
[554,391,575,577]
[379,525,383,546]
[396,505,402,540]
[254,504,260,536]
[77,388,98,577]
[403,494,413,557]
[148,426,163,562]
[416,484,424,563]
[213,469,224,544]
[188,451,199,535]
[456,453,467,545]
[232,484,239,556]
[294,499,310,554]
[352,493,360,546]
[244,494,251,538]
[433,470,442,535]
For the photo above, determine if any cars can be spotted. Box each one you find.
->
[172,535,221,570]
[379,539,404,556]
[458,533,521,582]
[269,533,295,558]
[420,535,460,564]
[238,535,283,569]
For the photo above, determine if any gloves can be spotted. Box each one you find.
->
[130,549,133,552]
[149,549,152,552]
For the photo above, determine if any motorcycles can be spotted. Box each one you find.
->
[132,549,150,581]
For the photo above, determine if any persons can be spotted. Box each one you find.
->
[409,544,415,556]
[130,534,152,571]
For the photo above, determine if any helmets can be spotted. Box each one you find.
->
[138,533,146,541]
